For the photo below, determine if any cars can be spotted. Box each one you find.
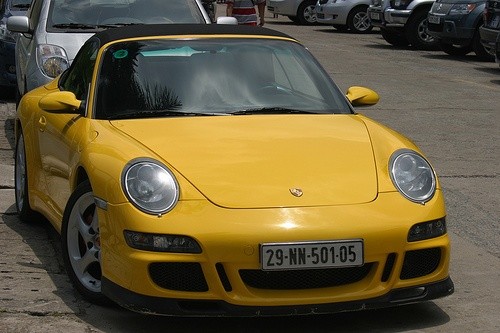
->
[426,0,496,63]
[315,0,373,32]
[367,0,442,51]
[0,0,239,112]
[479,0,500,70]
[266,0,320,23]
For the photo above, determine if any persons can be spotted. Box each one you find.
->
[228,50,267,104]
[226,0,266,27]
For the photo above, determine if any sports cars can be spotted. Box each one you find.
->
[14,23,455,319]
